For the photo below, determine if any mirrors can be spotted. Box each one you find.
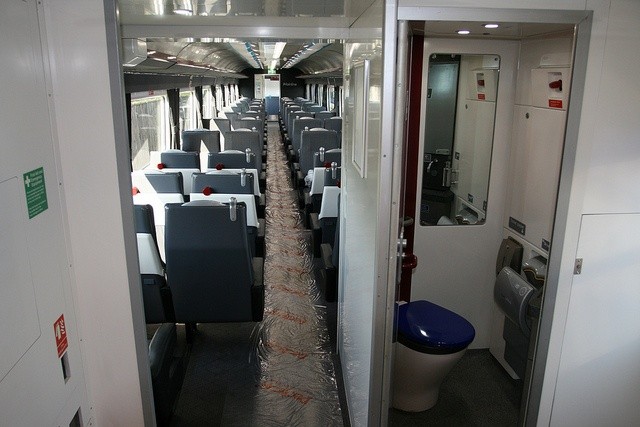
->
[418,52,503,227]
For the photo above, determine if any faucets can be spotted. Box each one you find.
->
[426,160,434,173]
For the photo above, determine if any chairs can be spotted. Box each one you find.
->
[279,96,347,301]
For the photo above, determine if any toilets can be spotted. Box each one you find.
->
[389,299,476,413]
